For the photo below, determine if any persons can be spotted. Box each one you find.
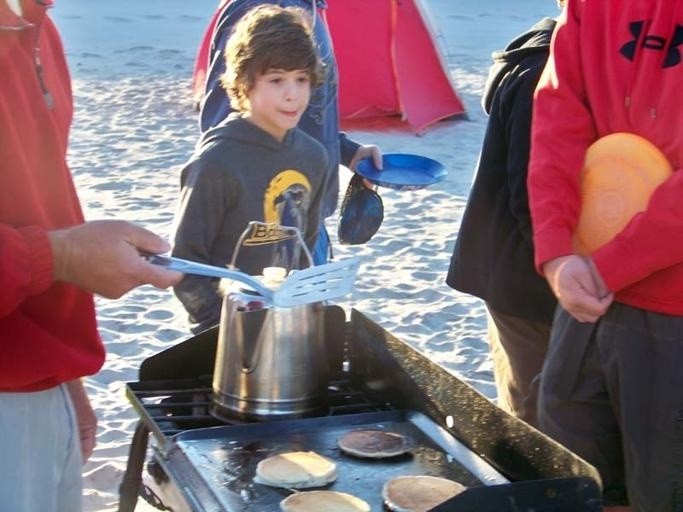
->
[530,1,683,511]
[448,0,579,435]
[173,3,330,333]
[197,0,386,269]
[1,2,189,512]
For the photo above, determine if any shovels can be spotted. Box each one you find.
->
[140,247,358,307]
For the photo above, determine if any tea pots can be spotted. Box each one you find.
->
[209,221,332,425]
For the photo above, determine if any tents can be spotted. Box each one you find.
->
[192,0,471,137]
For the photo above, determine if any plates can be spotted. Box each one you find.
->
[571,133,673,285]
[354,153,448,192]
